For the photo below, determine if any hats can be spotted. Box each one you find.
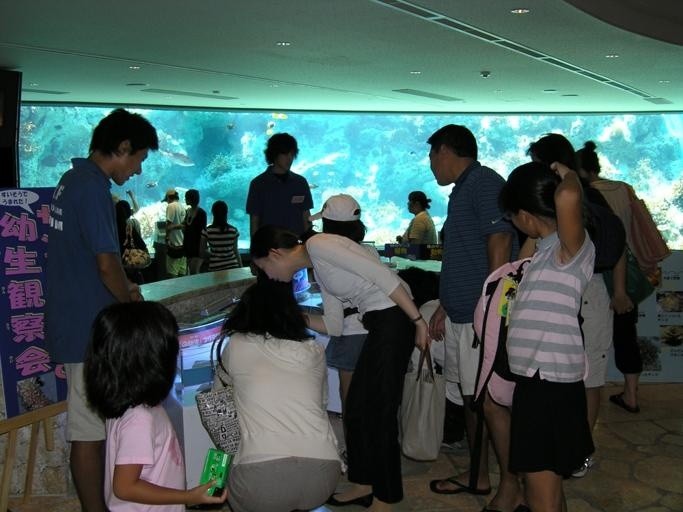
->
[308,194,360,222]
[161,189,178,202]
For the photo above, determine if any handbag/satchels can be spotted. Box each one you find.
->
[167,244,183,257]
[194,387,240,455]
[397,373,446,462]
[121,249,151,269]
[604,250,653,306]
[627,197,671,264]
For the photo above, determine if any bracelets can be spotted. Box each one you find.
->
[412,316,423,323]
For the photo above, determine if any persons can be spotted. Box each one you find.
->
[523,132,635,480]
[308,194,380,465]
[396,191,437,260]
[427,124,521,512]
[160,188,187,279]
[41,109,159,512]
[115,201,150,284]
[84,301,229,511]
[248,223,431,511]
[153,215,168,280]
[110,190,140,216]
[210,279,348,512]
[495,160,597,512]
[574,141,656,415]
[199,201,242,271]
[246,133,314,239]
[166,189,207,275]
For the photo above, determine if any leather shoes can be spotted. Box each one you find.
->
[326,492,373,508]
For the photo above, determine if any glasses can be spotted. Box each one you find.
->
[503,208,518,221]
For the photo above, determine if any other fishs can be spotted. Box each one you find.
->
[144,181,158,188]
[309,184,318,188]
[161,150,195,167]
[268,120,274,128]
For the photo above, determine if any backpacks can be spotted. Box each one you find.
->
[585,199,625,273]
[472,255,532,408]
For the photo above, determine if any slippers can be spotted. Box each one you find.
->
[610,392,639,412]
[429,477,490,496]
